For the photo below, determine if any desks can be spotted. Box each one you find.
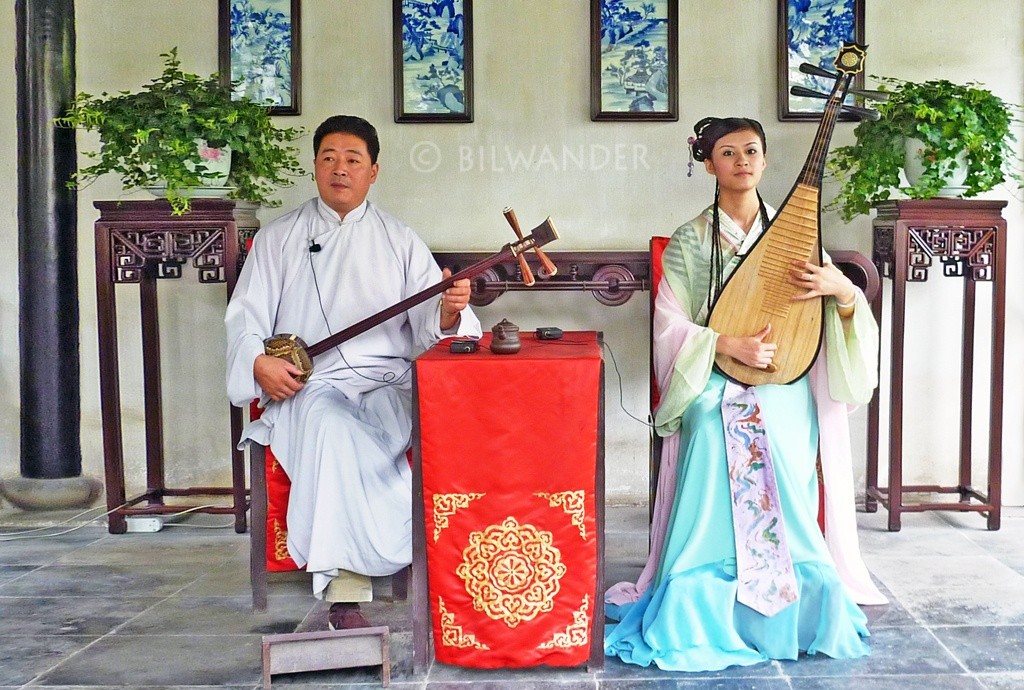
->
[864,199,1007,531]
[92,199,263,534]
[411,332,606,677]
[430,249,879,307]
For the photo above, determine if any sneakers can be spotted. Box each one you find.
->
[328,603,370,632]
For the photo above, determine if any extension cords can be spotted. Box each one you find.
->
[126,517,164,533]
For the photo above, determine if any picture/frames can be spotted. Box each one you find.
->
[218,0,302,115]
[776,0,866,122]
[590,0,679,122]
[392,0,474,124]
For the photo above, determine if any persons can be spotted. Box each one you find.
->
[223,115,483,631]
[602,117,889,672]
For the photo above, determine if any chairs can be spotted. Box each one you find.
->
[250,402,407,617]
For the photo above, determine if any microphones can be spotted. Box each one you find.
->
[310,244,321,252]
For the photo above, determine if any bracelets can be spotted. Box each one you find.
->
[836,290,857,307]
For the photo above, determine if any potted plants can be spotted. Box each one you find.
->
[819,74,1024,224]
[48,46,315,217]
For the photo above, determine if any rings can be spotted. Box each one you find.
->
[809,275,812,280]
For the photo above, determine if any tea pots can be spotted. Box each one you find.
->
[490,318,521,353]
